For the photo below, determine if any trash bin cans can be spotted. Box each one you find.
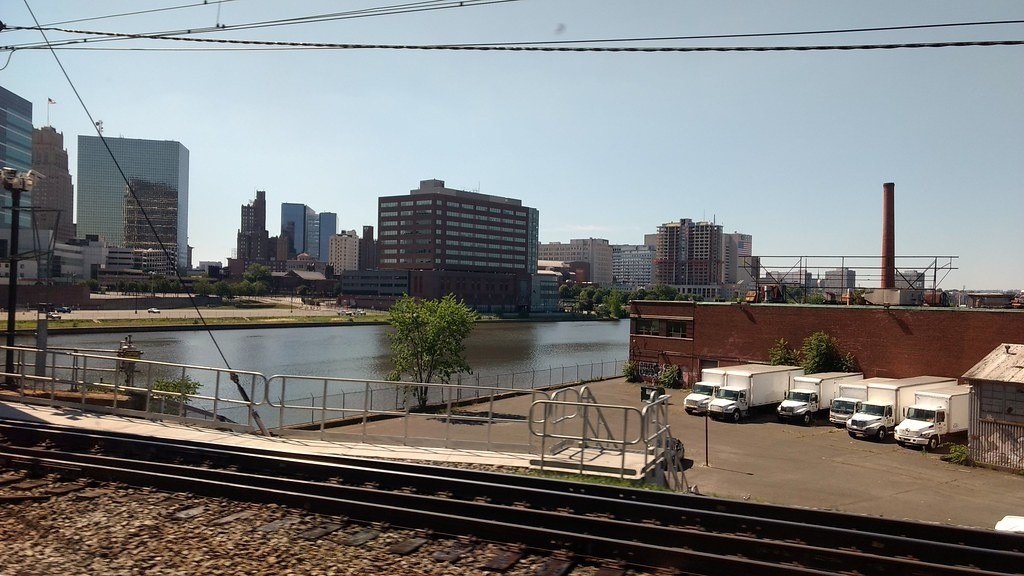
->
[640,386,666,403]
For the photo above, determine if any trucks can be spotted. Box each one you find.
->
[828,376,979,451]
[778,371,866,426]
[684,363,806,423]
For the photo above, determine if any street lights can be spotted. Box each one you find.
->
[0,166,48,390]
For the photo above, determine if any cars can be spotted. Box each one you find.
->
[47,311,62,320]
[655,438,686,470]
[337,310,353,317]
[54,306,72,313]
[353,309,366,316]
[148,308,160,313]
